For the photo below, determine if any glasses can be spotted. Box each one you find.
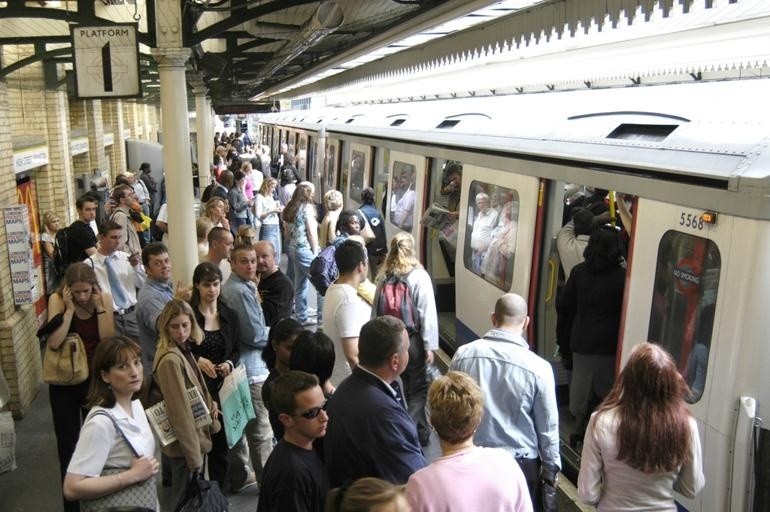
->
[288,401,329,419]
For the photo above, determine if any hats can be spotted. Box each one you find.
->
[139,162,150,170]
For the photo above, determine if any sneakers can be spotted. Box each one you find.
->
[231,472,258,493]
[292,303,317,326]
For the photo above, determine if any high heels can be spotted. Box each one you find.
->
[571,434,583,446]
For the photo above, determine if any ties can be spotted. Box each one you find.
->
[104,257,127,307]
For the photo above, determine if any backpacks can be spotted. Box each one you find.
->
[376,267,421,337]
[53,221,93,261]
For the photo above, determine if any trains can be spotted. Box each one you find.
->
[246,74,770,511]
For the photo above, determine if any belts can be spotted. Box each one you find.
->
[113,304,135,315]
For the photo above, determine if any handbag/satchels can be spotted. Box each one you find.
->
[218,362,257,450]
[147,375,164,407]
[79,467,157,512]
[42,332,89,385]
[310,234,350,296]
[173,471,228,512]
[360,221,376,245]
